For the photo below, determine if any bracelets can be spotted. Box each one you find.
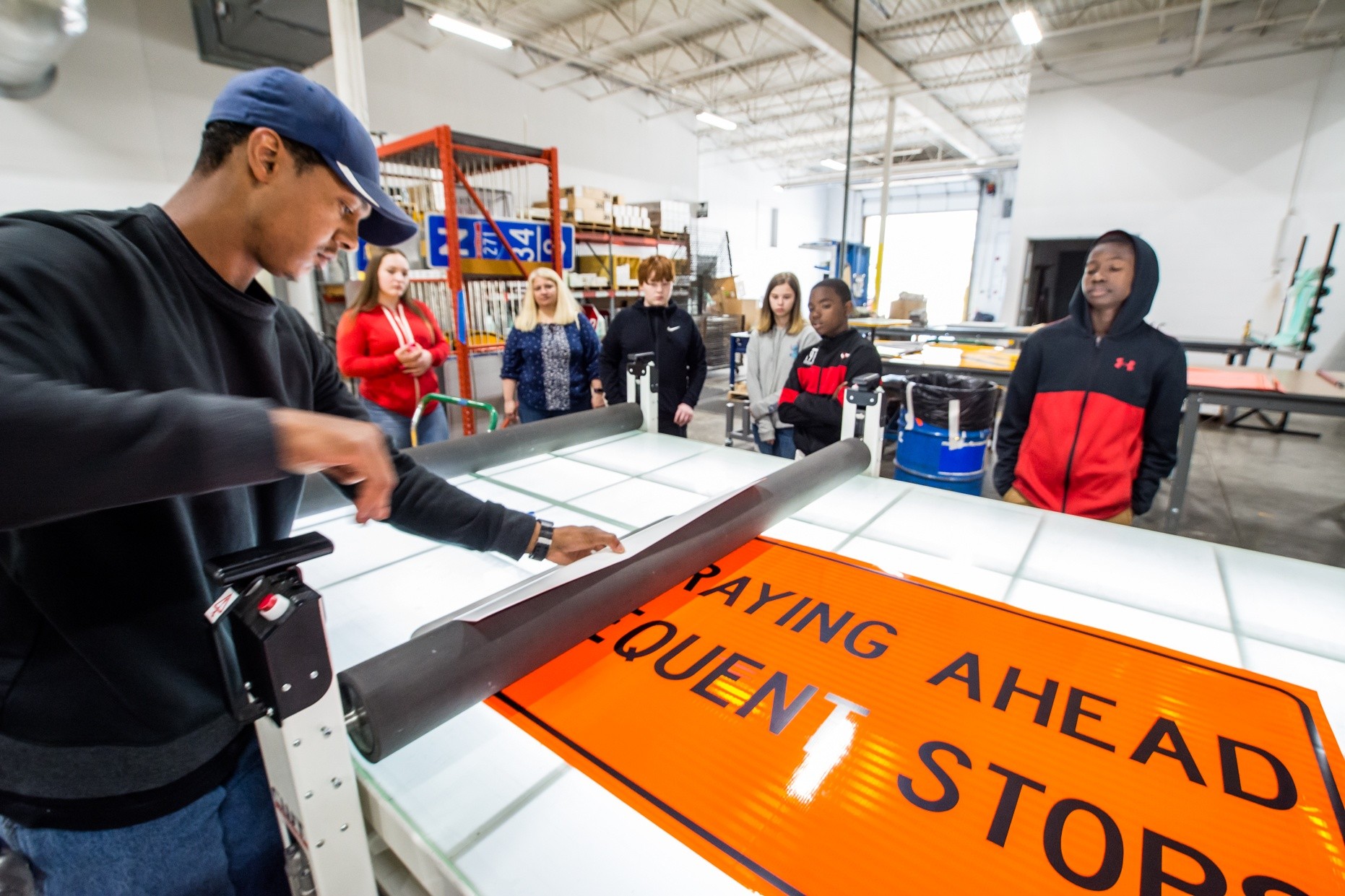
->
[529,520,553,562]
[594,388,604,393]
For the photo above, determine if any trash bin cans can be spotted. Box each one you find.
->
[894,371,1002,494]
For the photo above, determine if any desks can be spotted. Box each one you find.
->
[1163,333,1345,538]
[846,316,1037,386]
[727,328,757,399]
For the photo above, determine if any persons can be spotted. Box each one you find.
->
[0,65,624,896]
[336,247,449,449]
[778,278,883,457]
[992,230,1188,526]
[499,267,604,423]
[746,272,822,460]
[598,255,707,438]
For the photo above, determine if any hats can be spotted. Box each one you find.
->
[204,67,421,247]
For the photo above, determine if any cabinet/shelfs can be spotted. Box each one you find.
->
[546,231,701,327]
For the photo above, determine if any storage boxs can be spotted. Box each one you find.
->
[640,200,692,234]
[888,298,928,322]
[703,275,761,332]
[529,186,652,230]
